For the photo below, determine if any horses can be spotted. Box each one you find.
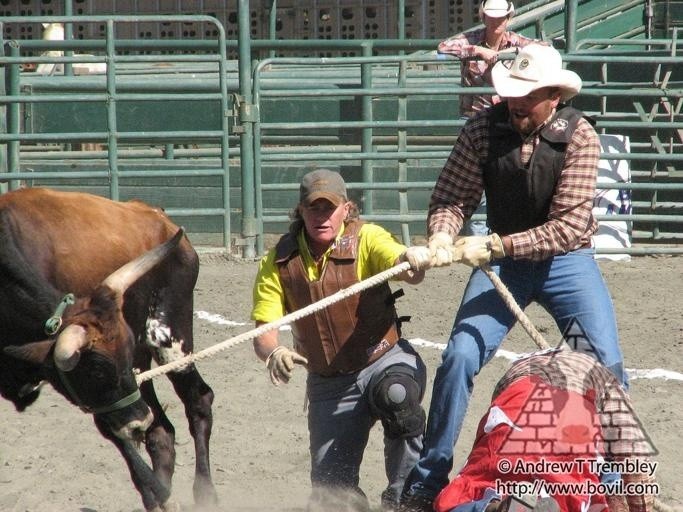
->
[34,22,106,72]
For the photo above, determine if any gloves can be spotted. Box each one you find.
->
[399,233,453,277]
[265,346,308,387]
[454,233,505,268]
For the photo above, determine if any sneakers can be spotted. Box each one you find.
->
[605,493,629,512]
[486,481,560,512]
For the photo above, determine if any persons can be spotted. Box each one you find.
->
[437,0,550,236]
[399,45,629,512]
[250,169,432,512]
[432,350,659,512]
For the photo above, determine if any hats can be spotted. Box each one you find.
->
[491,44,582,101]
[300,169,347,206]
[479,0,515,20]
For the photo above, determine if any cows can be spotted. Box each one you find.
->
[0,186,216,512]
